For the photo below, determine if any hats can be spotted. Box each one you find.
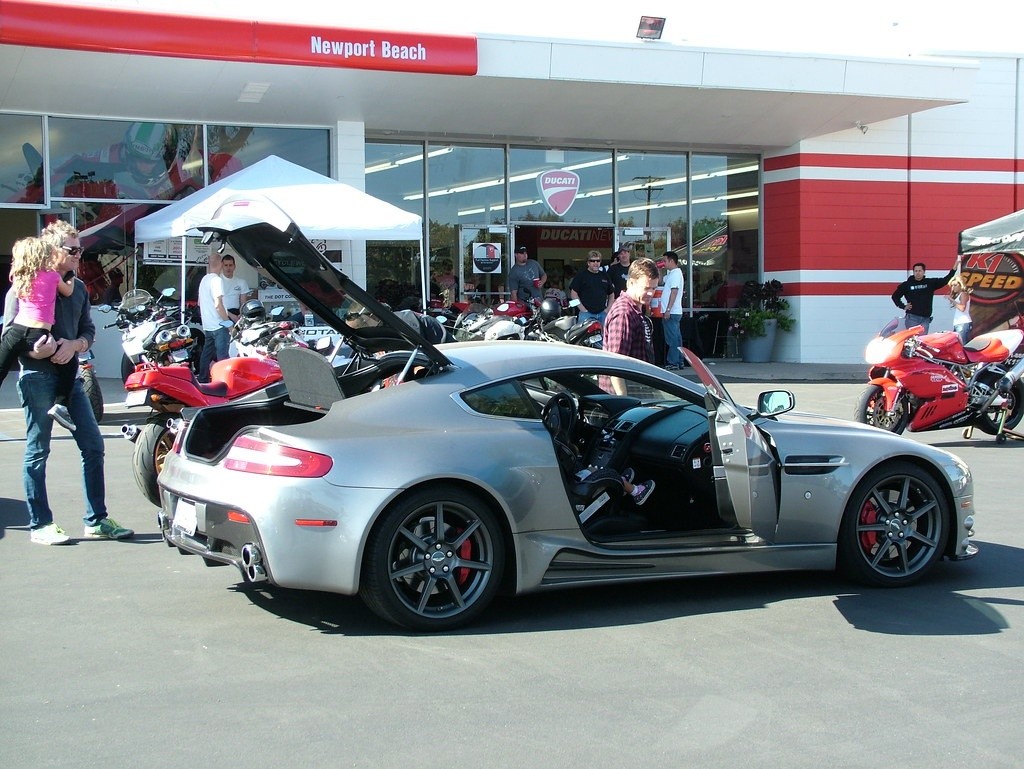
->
[514,245,528,253]
[618,245,630,254]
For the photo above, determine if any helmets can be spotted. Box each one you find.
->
[540,299,561,321]
[242,299,265,318]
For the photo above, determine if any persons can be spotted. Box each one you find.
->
[432,259,459,303]
[563,444,655,506]
[220,255,251,332]
[569,250,615,329]
[948,278,973,345]
[606,245,635,298]
[0,238,74,388]
[547,265,576,309]
[285,303,324,327]
[16,221,134,544]
[198,254,235,383]
[891,261,961,334]
[701,270,743,358]
[598,259,660,398]
[661,254,684,370]
[5,121,245,304]
[345,301,425,388]
[100,268,124,303]
[509,245,547,302]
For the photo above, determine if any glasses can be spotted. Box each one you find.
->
[588,260,600,262]
[519,251,528,254]
[61,245,85,255]
[122,274,124,276]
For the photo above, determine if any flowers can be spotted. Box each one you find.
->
[729,280,797,337]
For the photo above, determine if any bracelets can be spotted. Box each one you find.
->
[77,339,86,353]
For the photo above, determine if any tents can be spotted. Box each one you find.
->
[957,209,1024,336]
[134,155,426,324]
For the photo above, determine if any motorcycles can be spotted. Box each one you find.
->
[437,295,604,352]
[855,317,1024,447]
[120,335,332,508]
[219,299,312,363]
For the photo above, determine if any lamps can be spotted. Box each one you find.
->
[853,121,869,135]
[634,16,666,39]
[365,146,758,216]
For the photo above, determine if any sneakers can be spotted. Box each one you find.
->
[622,468,635,497]
[84,517,134,540]
[665,362,685,371]
[47,405,77,431]
[633,479,655,505]
[30,522,71,545]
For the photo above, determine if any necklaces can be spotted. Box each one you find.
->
[516,262,526,273]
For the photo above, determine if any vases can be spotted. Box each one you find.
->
[741,318,777,363]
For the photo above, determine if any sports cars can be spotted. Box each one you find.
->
[98,288,199,385]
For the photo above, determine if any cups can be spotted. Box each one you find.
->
[533,278,540,287]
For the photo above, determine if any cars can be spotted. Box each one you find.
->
[154,187,980,634]
